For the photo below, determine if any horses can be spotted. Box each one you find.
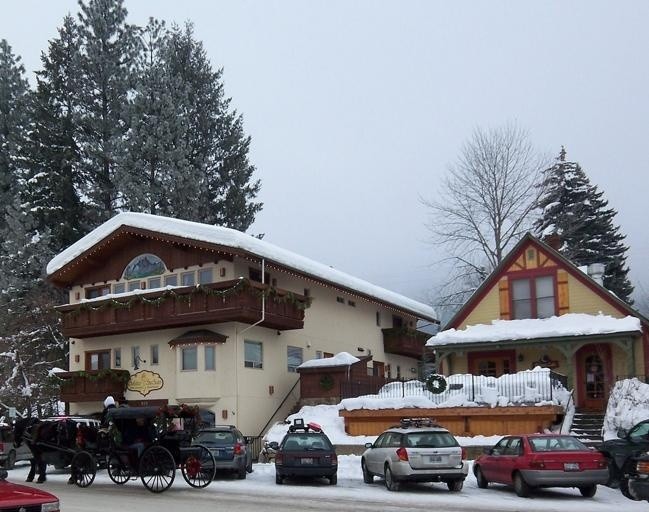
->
[13,417,90,485]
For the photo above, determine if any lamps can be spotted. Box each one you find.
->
[133,355,142,370]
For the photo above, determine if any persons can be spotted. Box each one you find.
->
[97,395,117,468]
[117,397,129,408]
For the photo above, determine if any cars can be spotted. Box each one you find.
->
[1,470,61,512]
[624,452,648,501]
[472,433,610,498]
[596,418,648,502]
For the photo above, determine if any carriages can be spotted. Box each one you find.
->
[11,401,218,494]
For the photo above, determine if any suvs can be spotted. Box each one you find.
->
[39,414,102,428]
[0,423,33,471]
[275,418,338,485]
[187,421,253,481]
[359,417,469,493]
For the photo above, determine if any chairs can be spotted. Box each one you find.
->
[408,435,440,445]
[313,442,322,447]
[287,439,298,446]
